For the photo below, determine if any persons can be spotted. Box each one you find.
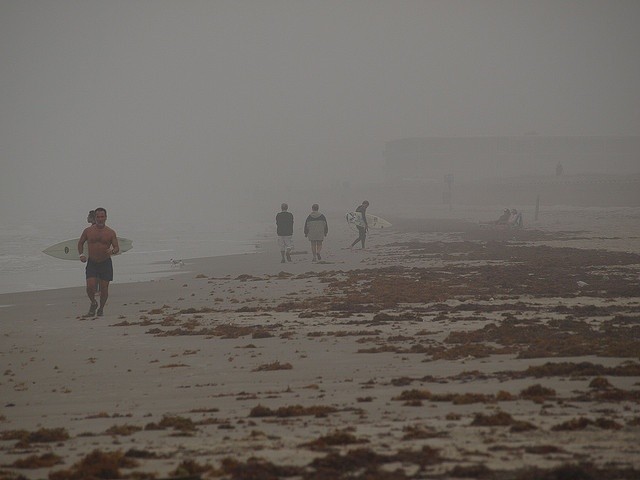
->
[275,203,294,262]
[304,204,328,262]
[86,210,95,225]
[478,207,518,225]
[78,207,120,315]
[348,200,370,250]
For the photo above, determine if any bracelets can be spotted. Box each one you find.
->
[79,253,86,258]
[112,249,115,254]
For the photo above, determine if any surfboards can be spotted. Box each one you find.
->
[343,212,392,229]
[42,237,133,260]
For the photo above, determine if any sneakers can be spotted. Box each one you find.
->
[97,309,103,317]
[286,252,291,262]
[281,259,285,263]
[316,252,321,260]
[312,258,316,261]
[89,301,98,317]
